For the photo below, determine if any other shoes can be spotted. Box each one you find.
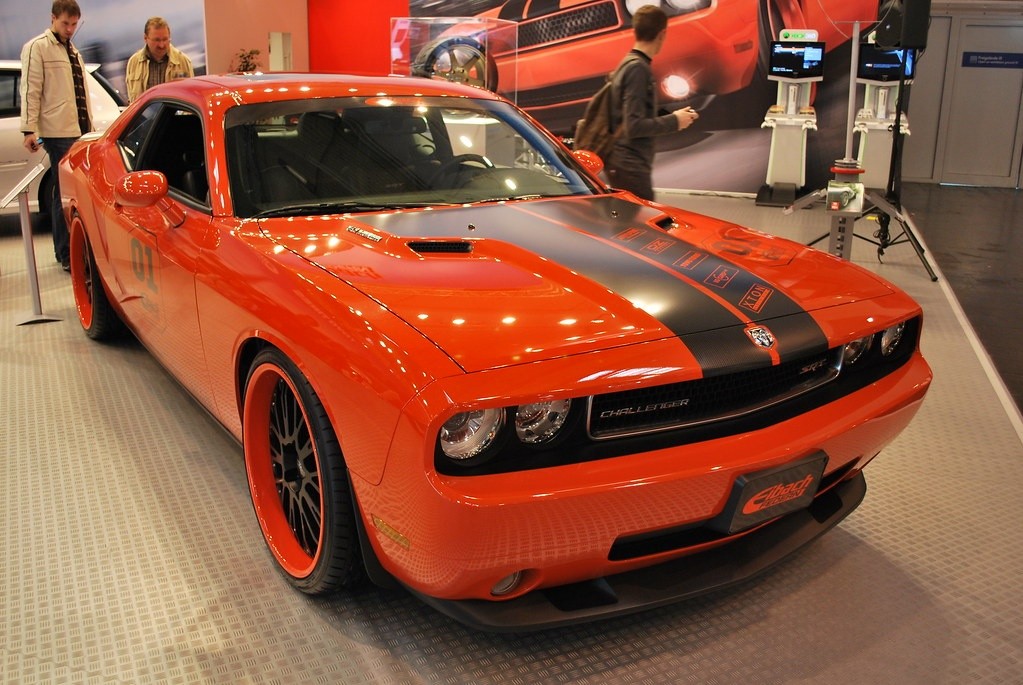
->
[62,265,71,271]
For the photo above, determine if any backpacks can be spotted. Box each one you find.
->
[573,56,653,159]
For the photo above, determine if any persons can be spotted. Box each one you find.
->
[601,4,699,204]
[19,0,96,274]
[126,16,194,104]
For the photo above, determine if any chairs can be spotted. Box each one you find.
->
[180,123,258,203]
[342,108,443,195]
[297,112,361,179]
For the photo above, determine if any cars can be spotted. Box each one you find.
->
[407,1,879,158]
[0,59,138,232]
[58,73,932,638]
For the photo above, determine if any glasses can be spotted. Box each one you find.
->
[145,35,171,42]
